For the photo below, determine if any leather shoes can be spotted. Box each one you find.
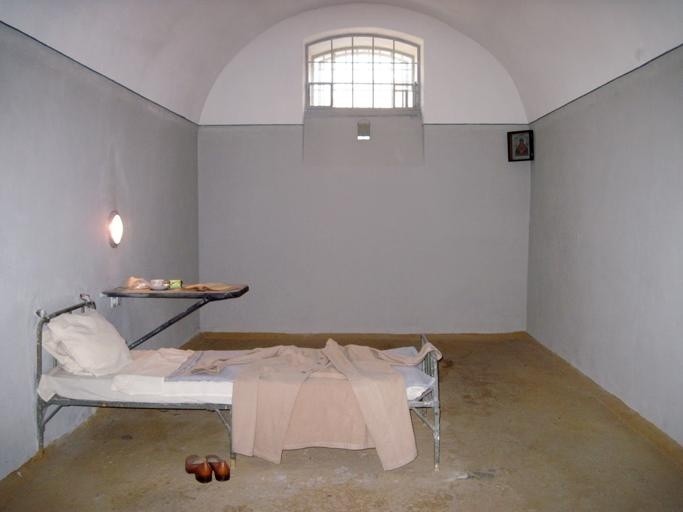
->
[206,454,230,482]
[185,454,213,483]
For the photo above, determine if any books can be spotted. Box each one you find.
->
[184,281,232,291]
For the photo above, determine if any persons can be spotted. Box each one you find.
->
[516,138,528,156]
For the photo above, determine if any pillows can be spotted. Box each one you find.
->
[38,306,133,377]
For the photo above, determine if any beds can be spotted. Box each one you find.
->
[28,293,442,469]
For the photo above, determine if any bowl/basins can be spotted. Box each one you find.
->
[150,279,170,290]
